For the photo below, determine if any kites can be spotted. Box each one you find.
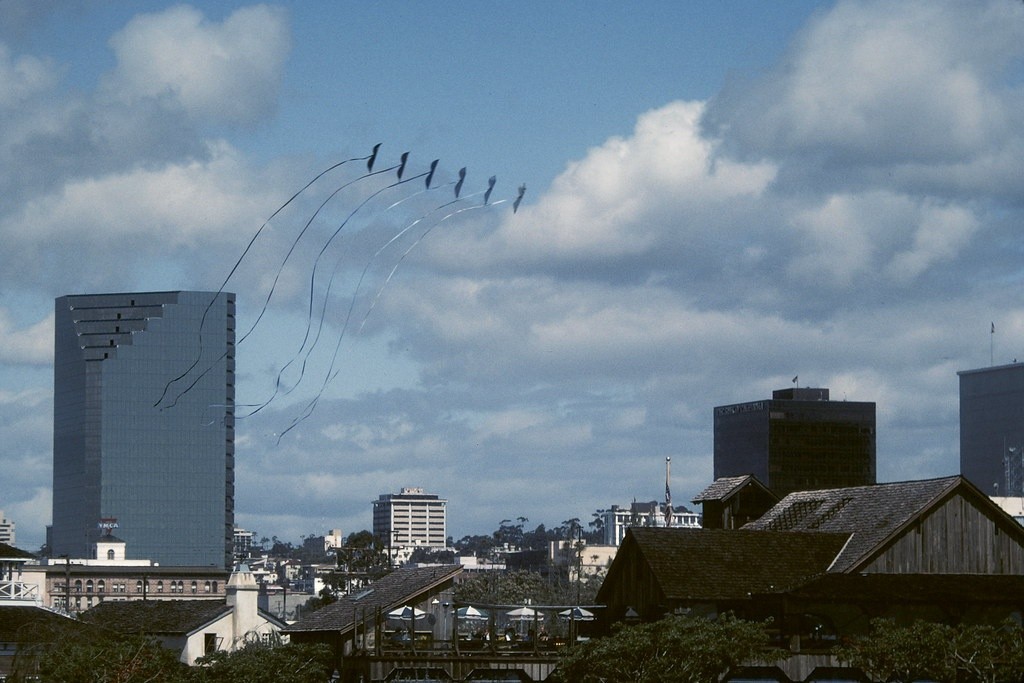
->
[365,142,527,215]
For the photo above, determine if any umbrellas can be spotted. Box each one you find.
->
[504,606,546,641]
[451,604,489,641]
[386,605,426,641]
[557,607,596,642]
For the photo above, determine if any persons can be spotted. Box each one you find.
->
[389,621,595,644]
